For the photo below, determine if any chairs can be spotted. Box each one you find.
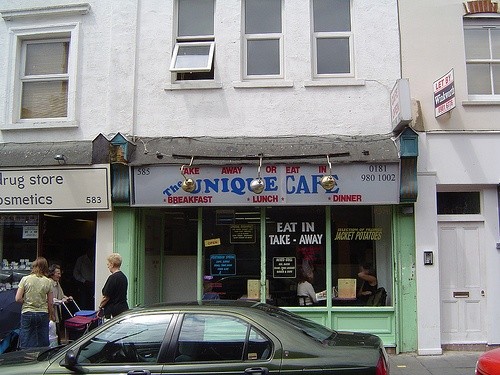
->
[175,340,199,361]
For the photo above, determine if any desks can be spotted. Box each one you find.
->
[240,295,274,304]
[316,297,357,305]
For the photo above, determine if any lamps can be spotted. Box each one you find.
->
[250,158,265,194]
[319,155,334,191]
[54,153,67,165]
[180,156,195,192]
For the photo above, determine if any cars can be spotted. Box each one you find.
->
[2,298,391,375]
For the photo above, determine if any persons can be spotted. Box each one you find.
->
[48,263,74,345]
[355,262,379,305]
[14,255,56,349]
[97,253,130,320]
[74,243,96,309]
[296,271,322,306]
